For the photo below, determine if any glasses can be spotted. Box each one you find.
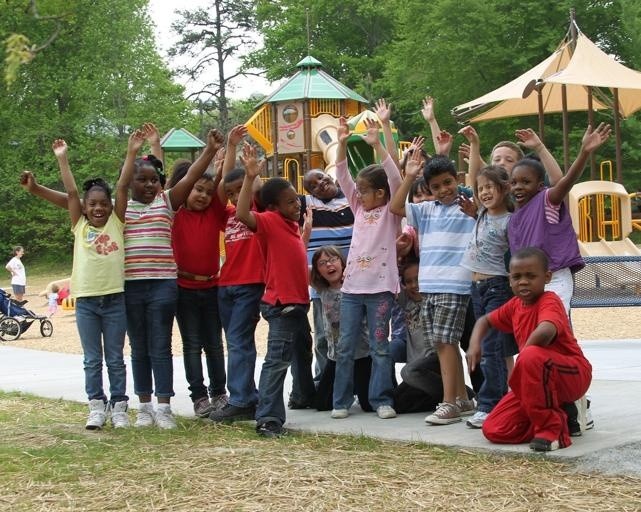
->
[319,256,339,266]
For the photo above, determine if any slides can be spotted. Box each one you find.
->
[578,238,641,287]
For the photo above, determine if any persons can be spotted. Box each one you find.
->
[17,96,615,452]
[5,246,27,304]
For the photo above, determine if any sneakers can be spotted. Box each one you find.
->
[134,402,155,427]
[108,399,131,429]
[377,404,395,418]
[194,396,215,417]
[210,403,256,422]
[212,393,229,409]
[425,394,489,428]
[331,406,349,418]
[86,398,111,430]
[153,403,177,430]
[256,420,283,436]
[529,398,594,451]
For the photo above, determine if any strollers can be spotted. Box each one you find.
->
[0,290,54,341]
